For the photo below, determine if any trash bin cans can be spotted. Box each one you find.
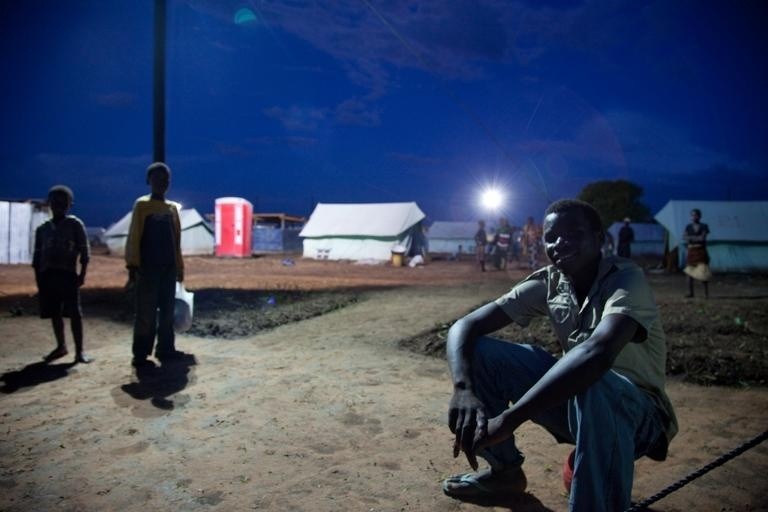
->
[391,251,405,267]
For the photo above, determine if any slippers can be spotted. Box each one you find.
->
[440,471,525,500]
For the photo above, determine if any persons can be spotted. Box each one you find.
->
[475,216,635,271]
[31,185,94,363]
[442,198,678,512]
[681,209,713,299]
[125,161,184,368]
